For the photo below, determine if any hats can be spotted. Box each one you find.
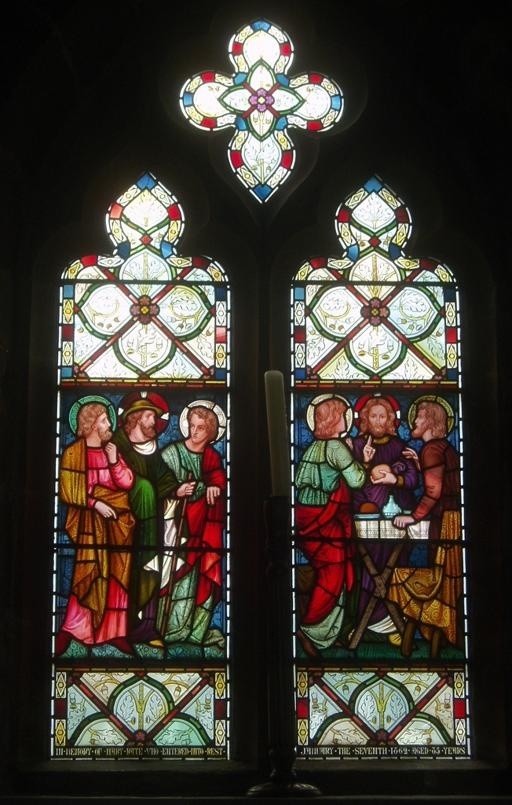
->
[122,400,162,421]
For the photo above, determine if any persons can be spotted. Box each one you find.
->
[110,389,197,653]
[384,393,465,651]
[291,391,367,653]
[52,394,136,656]
[344,391,422,651]
[157,398,228,650]
